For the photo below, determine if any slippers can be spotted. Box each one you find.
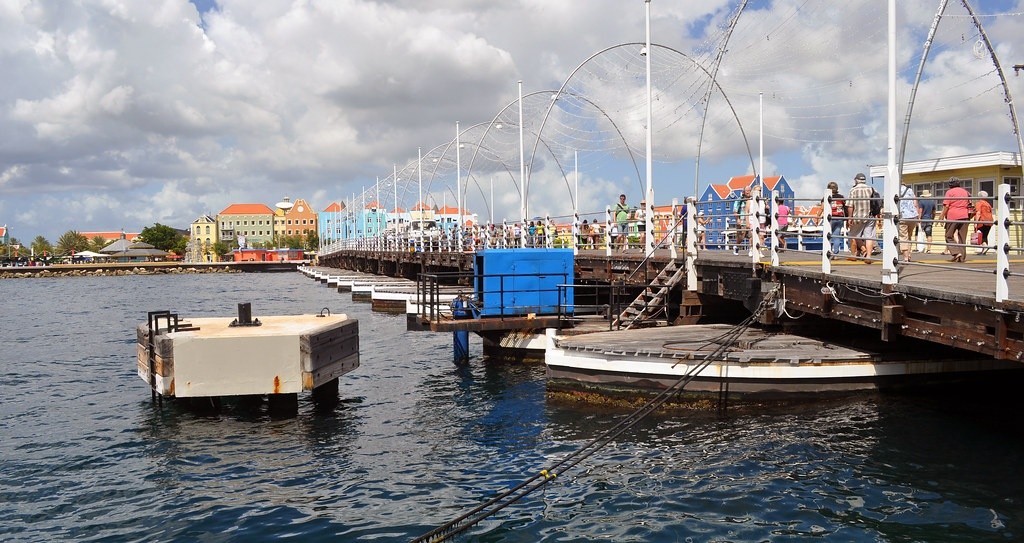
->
[941,252,949,255]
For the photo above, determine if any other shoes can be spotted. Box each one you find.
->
[846,249,882,264]
[733,242,788,258]
[978,248,988,255]
[911,250,931,254]
[831,256,840,260]
[696,247,708,251]
[948,254,965,262]
[640,248,646,254]
[615,245,628,254]
[900,256,911,265]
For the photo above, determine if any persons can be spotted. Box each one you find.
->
[938,177,972,262]
[697,211,711,251]
[590,219,601,249]
[634,200,646,247]
[613,194,631,249]
[745,185,770,258]
[579,220,590,249]
[845,173,882,264]
[918,190,936,253]
[973,191,993,255]
[679,197,688,249]
[898,181,918,264]
[733,186,753,255]
[777,197,790,252]
[571,217,582,249]
[359,219,556,250]
[820,180,848,260]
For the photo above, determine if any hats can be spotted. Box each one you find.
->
[698,212,704,216]
[854,173,866,180]
[920,190,931,198]
[640,200,646,203]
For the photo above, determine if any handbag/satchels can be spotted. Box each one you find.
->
[970,228,982,245]
[871,187,883,216]
[765,208,771,225]
[968,192,975,219]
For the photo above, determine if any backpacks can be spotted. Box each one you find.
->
[738,197,743,218]
[832,194,844,217]
[590,225,595,236]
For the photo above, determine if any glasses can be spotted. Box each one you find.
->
[620,198,625,200]
